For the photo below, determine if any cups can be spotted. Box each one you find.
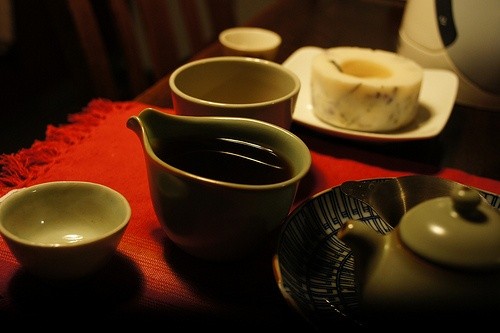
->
[168,56,301,130]
[126,108,311,257]
[219,28,282,61]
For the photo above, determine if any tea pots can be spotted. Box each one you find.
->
[338,187,500,332]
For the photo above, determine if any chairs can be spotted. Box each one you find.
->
[66,0,231,102]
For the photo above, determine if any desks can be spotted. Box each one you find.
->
[0,0,500,333]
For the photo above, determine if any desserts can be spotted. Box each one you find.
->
[311,47,423,132]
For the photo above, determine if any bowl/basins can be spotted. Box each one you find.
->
[0,180,132,289]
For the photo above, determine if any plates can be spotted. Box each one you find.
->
[283,46,459,140]
[272,177,500,332]
